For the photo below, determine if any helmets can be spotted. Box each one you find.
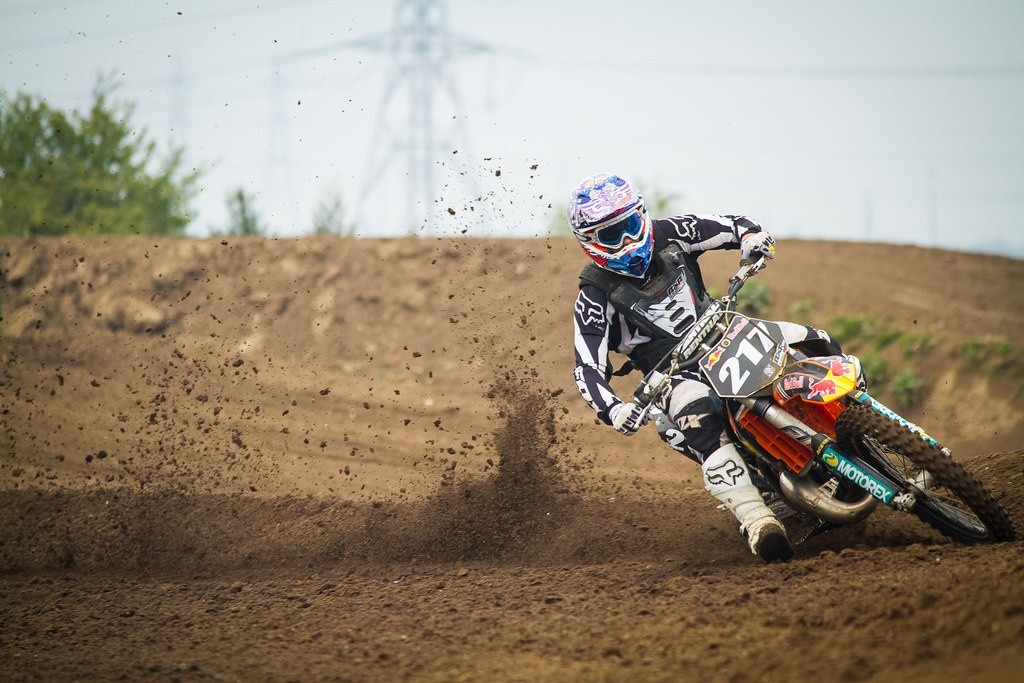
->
[568,174,654,278]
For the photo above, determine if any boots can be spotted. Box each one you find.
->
[701,443,793,562]
[843,352,868,395]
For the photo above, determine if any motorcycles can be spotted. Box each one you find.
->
[618,248,1019,550]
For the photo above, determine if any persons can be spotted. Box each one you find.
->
[567,174,868,566]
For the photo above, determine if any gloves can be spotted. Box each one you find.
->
[609,403,653,436]
[741,230,775,270]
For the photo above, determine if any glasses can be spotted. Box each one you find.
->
[591,207,645,250]
